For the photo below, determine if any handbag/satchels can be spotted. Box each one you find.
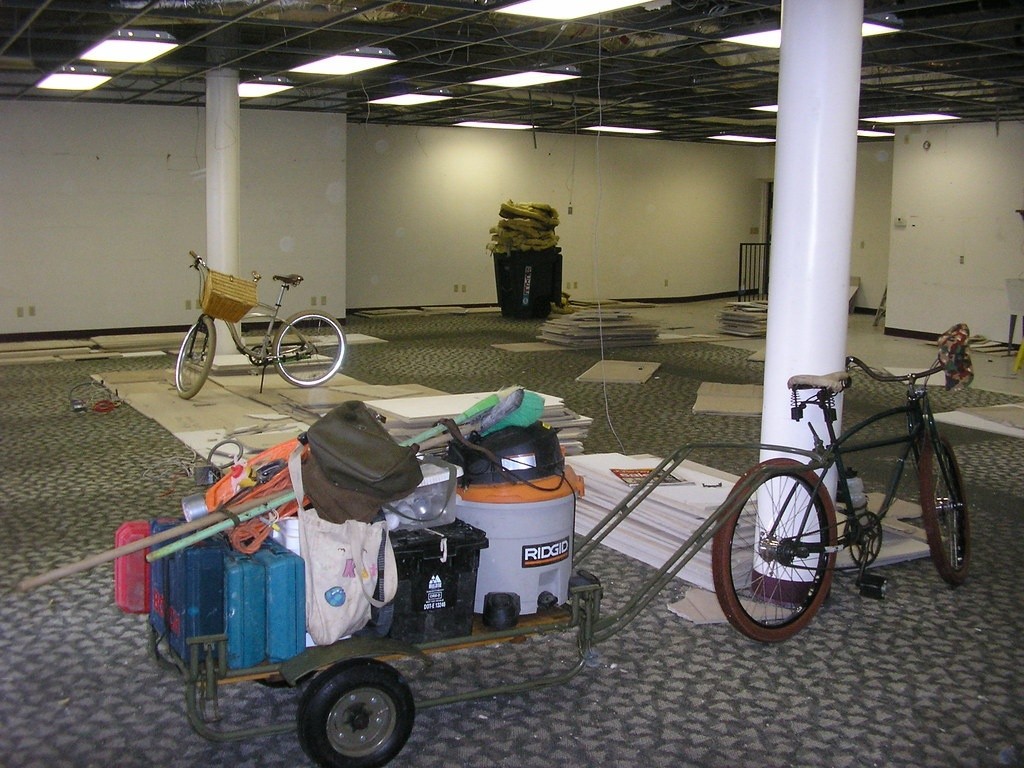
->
[302,400,457,524]
[288,445,399,646]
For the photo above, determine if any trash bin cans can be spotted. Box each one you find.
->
[493,247,563,321]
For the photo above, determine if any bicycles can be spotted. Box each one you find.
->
[712,334,990,645]
[174,249,349,400]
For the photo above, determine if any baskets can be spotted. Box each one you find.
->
[203,270,257,323]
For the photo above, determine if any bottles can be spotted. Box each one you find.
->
[845,466,871,526]
